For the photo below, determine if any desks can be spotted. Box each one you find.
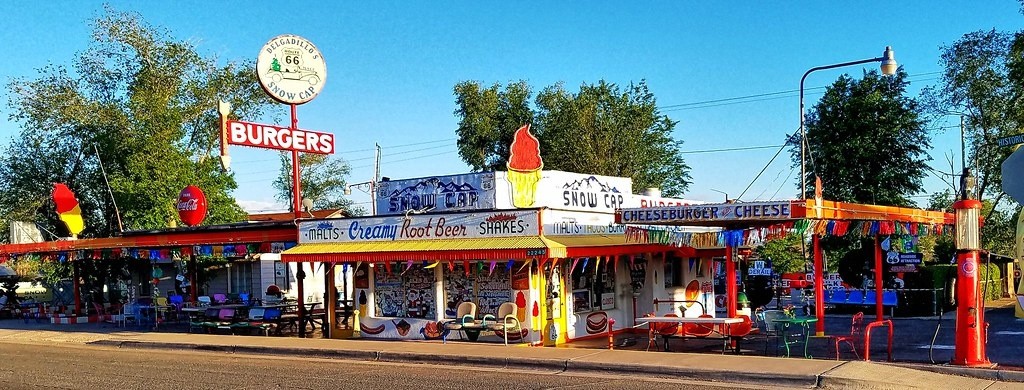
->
[181,304,245,333]
[635,317,745,355]
[336,299,354,308]
[772,318,818,359]
[254,302,322,330]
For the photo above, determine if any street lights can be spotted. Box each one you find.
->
[950,167,997,368]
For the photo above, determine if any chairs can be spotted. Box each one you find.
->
[652,314,679,351]
[1,293,285,336]
[845,291,863,305]
[862,292,876,306]
[685,315,714,338]
[436,302,476,344]
[883,291,897,316]
[728,315,751,354]
[829,312,864,362]
[477,302,523,346]
[830,290,846,304]
[824,290,829,304]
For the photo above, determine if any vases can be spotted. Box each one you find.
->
[787,314,796,319]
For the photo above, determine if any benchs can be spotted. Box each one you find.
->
[278,313,325,322]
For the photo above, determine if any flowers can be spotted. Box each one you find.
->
[784,304,795,317]
